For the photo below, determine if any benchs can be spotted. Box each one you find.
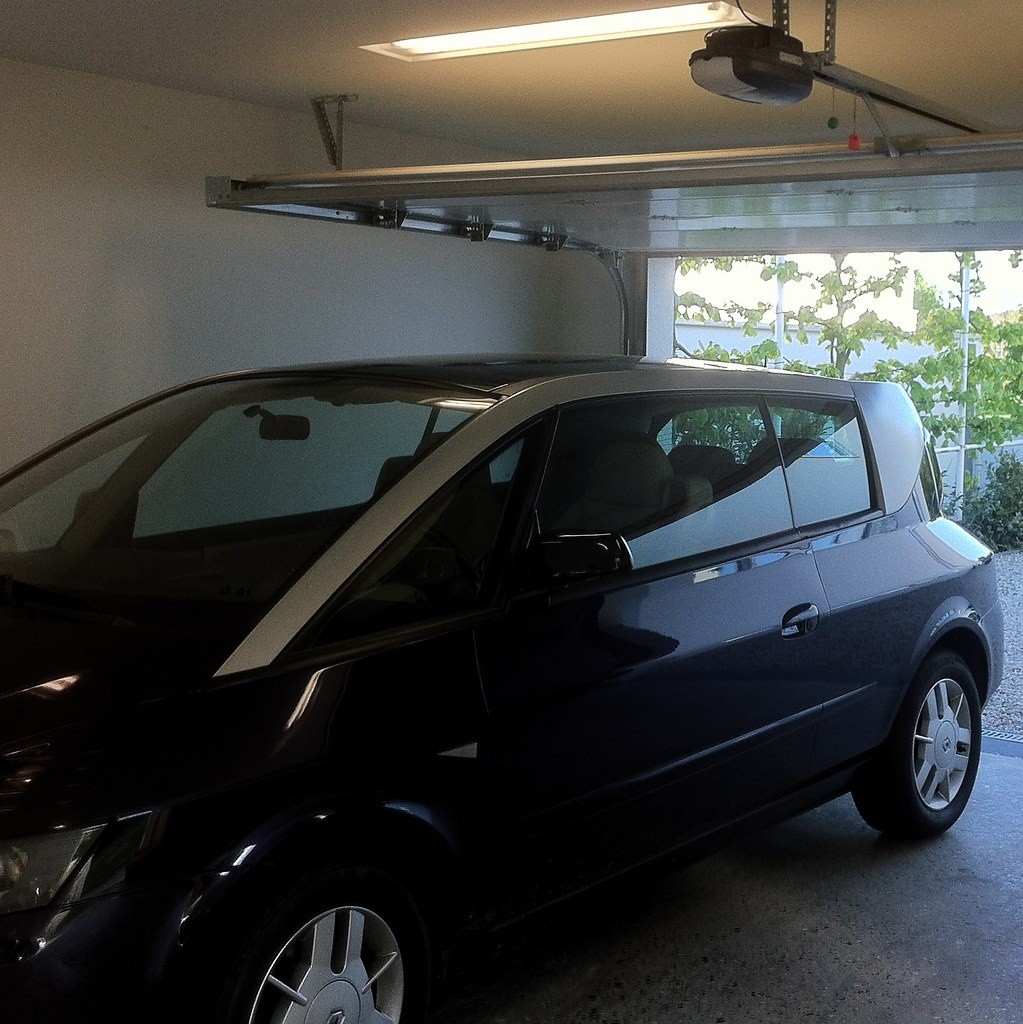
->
[652,438,822,517]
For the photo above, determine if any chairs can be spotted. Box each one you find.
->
[374,432,492,612]
[544,433,674,549]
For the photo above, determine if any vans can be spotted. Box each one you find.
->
[1,354,1004,1023]
